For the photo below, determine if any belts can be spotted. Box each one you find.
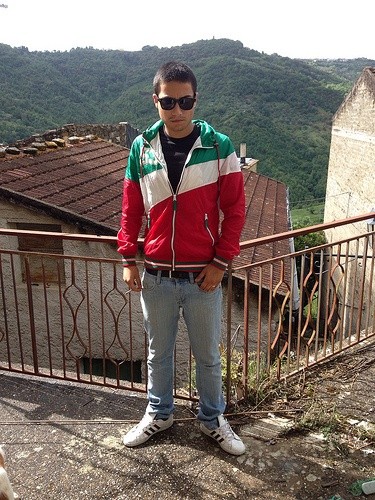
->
[146,269,206,279]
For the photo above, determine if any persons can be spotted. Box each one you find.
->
[116,62,246,455]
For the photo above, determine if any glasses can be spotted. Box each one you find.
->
[158,98,196,111]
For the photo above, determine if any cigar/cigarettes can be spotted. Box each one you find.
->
[125,288,132,295]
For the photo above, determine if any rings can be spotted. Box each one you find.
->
[212,285,216,289]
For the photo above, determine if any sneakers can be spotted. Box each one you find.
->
[123,414,173,447]
[200,414,245,455]
